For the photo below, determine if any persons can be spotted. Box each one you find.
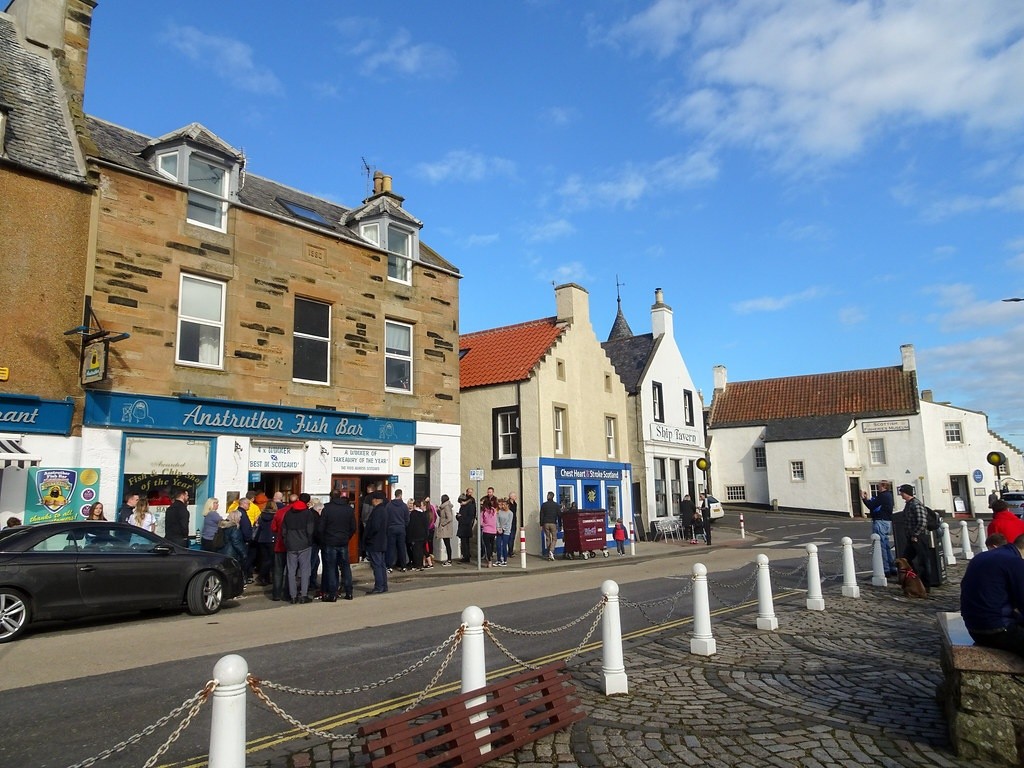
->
[456,489,476,562]
[681,493,710,543]
[165,490,190,547]
[202,490,356,604]
[862,484,897,576]
[85,502,109,547]
[436,494,453,566]
[613,518,628,555]
[961,501,1024,652]
[898,483,930,591]
[480,487,517,567]
[1,517,22,530]
[358,483,437,594]
[988,486,1007,508]
[540,492,577,560]
[116,495,156,545]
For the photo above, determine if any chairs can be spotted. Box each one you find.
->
[63,533,85,552]
[654,519,685,543]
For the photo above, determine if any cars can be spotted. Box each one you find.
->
[707,494,725,524]
[0,520,244,643]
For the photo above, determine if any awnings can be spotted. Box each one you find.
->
[0,440,41,468]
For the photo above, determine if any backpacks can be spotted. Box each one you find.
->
[926,507,942,530]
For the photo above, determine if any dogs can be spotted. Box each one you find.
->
[890,557,928,600]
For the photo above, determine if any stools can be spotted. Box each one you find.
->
[935,610,1024,768]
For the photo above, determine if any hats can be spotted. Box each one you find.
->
[370,490,386,500]
[899,485,914,497]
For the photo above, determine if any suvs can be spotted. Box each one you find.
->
[1002,492,1024,520]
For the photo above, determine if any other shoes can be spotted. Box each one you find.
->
[498,562,508,567]
[618,553,625,557]
[493,561,502,566]
[460,556,470,562]
[548,552,556,562]
[442,561,452,566]
[323,595,337,603]
[256,577,268,586]
[344,594,353,600]
[273,595,281,601]
[289,598,299,604]
[367,589,384,596]
[386,564,434,573]
[301,596,312,603]
